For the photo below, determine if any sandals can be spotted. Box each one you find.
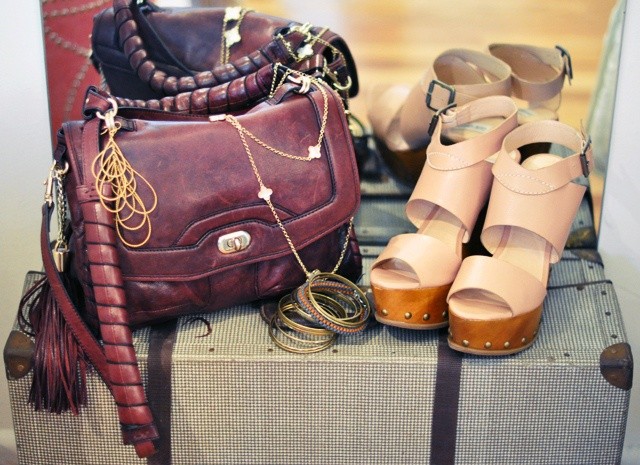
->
[446,118,594,359]
[363,48,513,183]
[367,95,521,332]
[484,34,574,165]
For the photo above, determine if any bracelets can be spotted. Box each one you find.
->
[269,273,371,354]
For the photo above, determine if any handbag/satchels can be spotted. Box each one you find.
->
[79,2,360,110]
[32,67,354,459]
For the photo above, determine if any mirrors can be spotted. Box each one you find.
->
[37,0,628,251]
